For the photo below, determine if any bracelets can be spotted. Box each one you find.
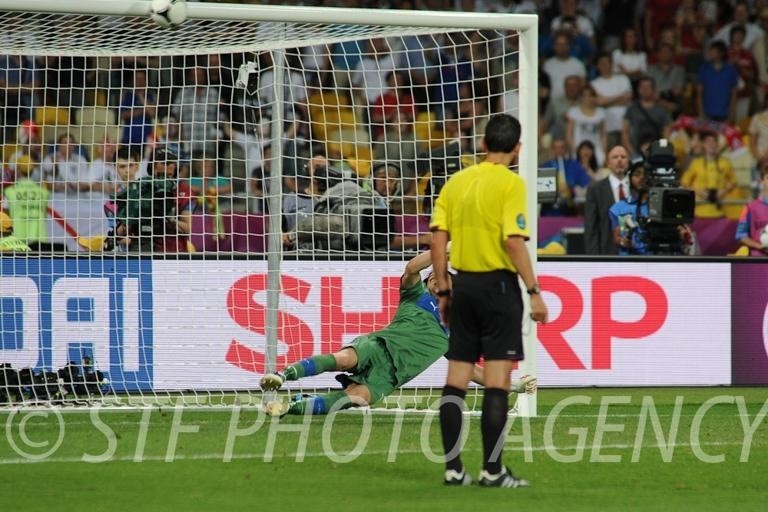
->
[526,284,541,295]
[436,288,452,299]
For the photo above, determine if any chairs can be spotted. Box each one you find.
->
[443,465,477,487]
[259,371,285,392]
[265,401,289,417]
[478,464,529,488]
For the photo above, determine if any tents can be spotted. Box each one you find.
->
[302,151,321,177]
[146,145,171,175]
[367,162,399,185]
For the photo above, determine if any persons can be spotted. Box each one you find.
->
[427,111,550,487]
[0,1,768,263]
[256,249,538,419]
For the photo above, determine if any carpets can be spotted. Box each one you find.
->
[619,184,626,200]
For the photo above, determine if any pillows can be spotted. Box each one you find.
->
[147,146,184,178]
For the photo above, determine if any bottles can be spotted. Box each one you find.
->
[16,155,36,174]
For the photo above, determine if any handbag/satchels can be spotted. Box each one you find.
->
[526,284,541,295]
[436,288,452,299]
